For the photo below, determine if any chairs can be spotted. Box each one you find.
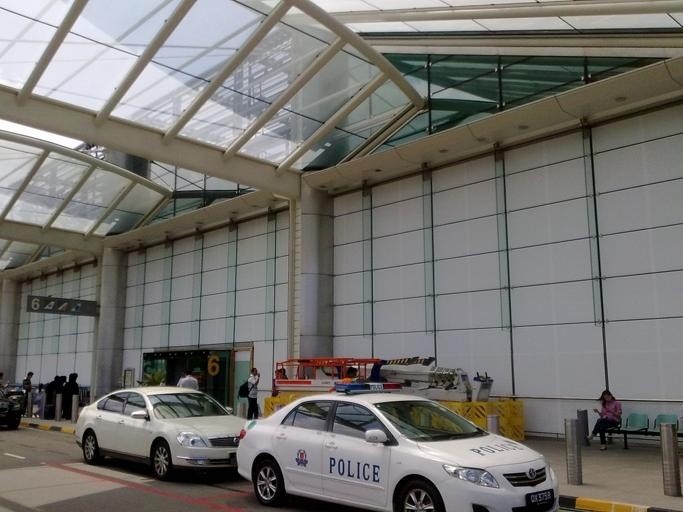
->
[605,413,682,450]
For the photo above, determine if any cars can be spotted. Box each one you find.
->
[236,383,560,512]
[0,390,21,429]
[74,386,247,480]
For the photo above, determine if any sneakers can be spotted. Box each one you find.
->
[588,434,607,450]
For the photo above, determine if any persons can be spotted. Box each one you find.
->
[176,367,199,391]
[247,368,260,419]
[584,388,623,451]
[0,371,90,420]
[280,368,287,379]
[328,367,358,392]
[271,371,282,411]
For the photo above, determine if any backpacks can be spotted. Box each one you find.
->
[237,375,258,398]
